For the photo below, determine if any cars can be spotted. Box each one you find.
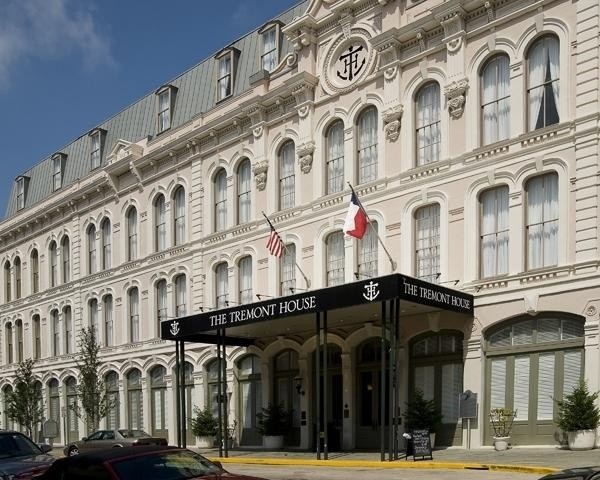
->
[0,429,58,479]
[44,446,268,479]
[62,430,168,456]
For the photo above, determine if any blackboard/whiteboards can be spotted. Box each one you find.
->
[458,390,478,418]
[406,429,432,457]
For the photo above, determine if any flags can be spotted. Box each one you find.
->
[343,192,367,239]
[266,229,285,259]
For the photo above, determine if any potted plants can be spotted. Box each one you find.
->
[254,399,297,449]
[489,407,518,452]
[188,405,238,449]
[404,392,445,450]
[549,381,600,451]
